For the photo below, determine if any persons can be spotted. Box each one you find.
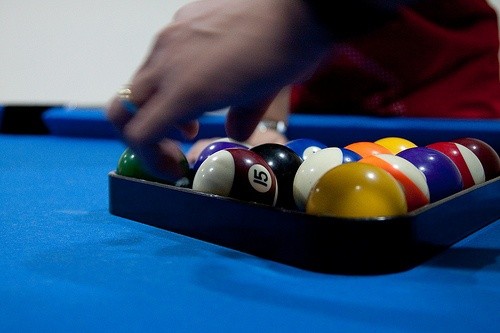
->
[107,1,500,177]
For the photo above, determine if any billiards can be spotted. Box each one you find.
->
[116,138,191,188]
[193,140,304,208]
[284,137,500,217]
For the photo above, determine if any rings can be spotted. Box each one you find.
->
[120,86,137,113]
